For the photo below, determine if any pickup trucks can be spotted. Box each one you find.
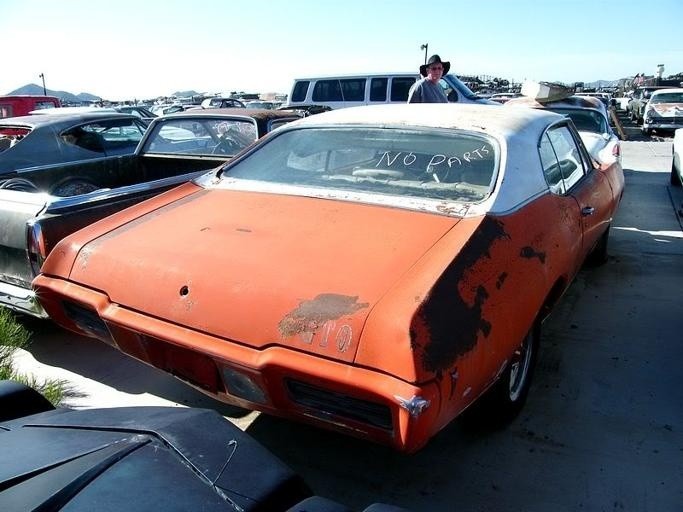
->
[0,107,315,326]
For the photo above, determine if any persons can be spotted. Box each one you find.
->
[406,54,450,104]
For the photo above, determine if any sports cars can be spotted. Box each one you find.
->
[30,101,625,457]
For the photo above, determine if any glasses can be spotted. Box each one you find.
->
[429,67,444,71]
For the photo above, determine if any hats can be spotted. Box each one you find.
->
[419,54,451,78]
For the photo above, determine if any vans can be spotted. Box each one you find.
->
[284,72,501,111]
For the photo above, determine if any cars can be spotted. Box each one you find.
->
[0,378,423,512]
[464,79,521,104]
[0,90,294,116]
[564,82,682,135]
[669,123,683,189]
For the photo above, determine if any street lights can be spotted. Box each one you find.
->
[420,43,427,66]
